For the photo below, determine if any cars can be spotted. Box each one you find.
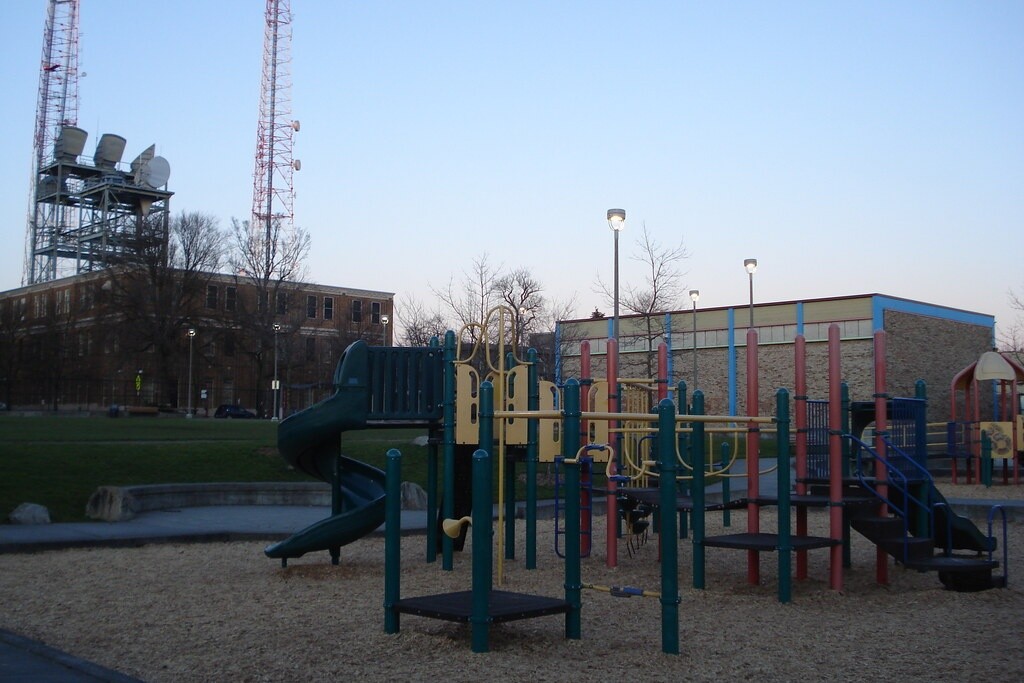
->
[214,404,255,419]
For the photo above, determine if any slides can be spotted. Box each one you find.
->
[263,388,390,559]
[891,480,997,551]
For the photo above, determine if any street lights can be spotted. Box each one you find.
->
[607,208,626,378]
[186,328,196,414]
[518,304,527,361]
[744,258,758,331]
[272,322,282,417]
[688,289,699,391]
[380,314,390,346]
